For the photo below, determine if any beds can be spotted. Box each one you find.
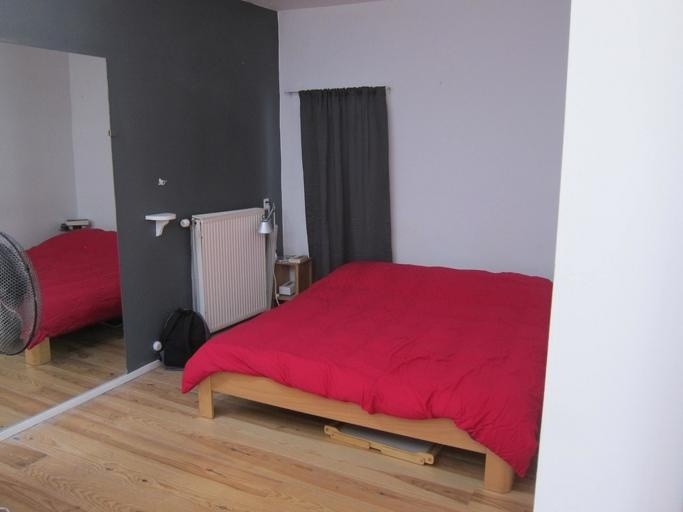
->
[22,228,122,365]
[182,260,552,495]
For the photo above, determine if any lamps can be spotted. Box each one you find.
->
[258,201,276,233]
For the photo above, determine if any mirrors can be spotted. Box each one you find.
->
[0,41,126,443]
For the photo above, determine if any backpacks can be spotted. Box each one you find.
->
[153,307,212,371]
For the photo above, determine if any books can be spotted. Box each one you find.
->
[65,219,89,226]
[276,254,291,262]
[287,254,307,263]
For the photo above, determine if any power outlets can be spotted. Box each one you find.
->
[264,199,270,210]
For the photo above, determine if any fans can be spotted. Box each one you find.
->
[0,231,41,355]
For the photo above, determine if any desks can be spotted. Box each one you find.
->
[274,255,310,302]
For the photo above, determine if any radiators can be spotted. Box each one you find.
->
[180,207,267,334]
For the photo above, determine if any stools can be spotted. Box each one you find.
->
[66,219,88,230]
